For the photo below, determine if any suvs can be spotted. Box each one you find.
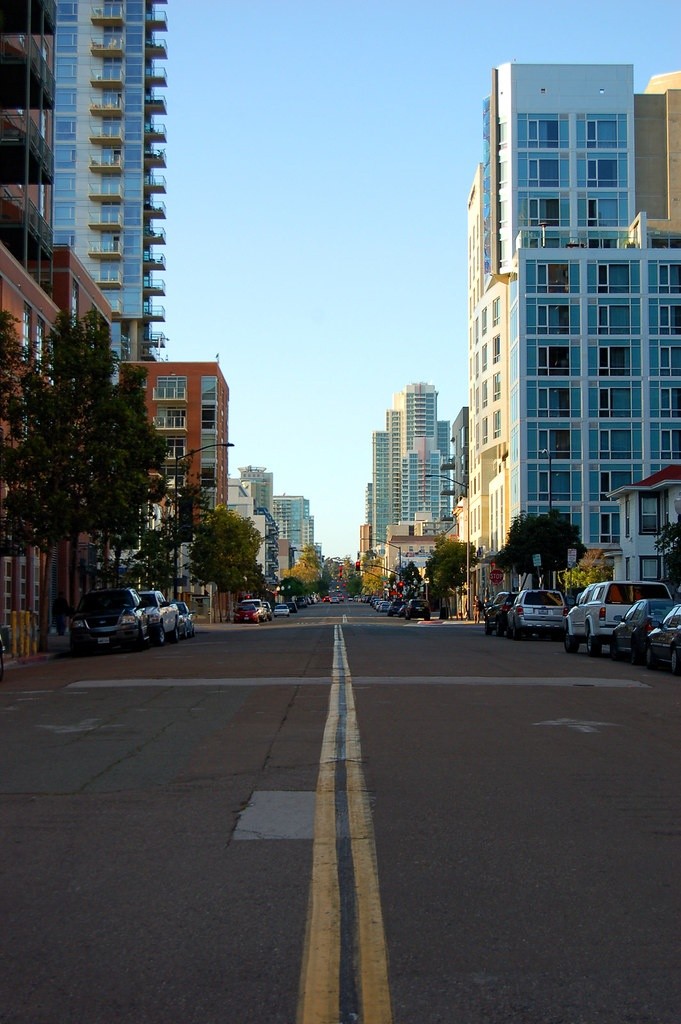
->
[239,599,268,622]
[506,589,568,642]
[563,580,673,658]
[404,598,432,620]
[136,590,180,647]
[483,591,520,637]
[66,586,152,656]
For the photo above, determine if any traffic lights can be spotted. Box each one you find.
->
[397,582,403,593]
[356,562,360,571]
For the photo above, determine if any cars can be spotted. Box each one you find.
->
[284,602,297,613]
[273,604,291,617]
[610,598,681,666]
[169,601,197,640]
[232,603,260,624]
[294,590,409,618]
[328,595,340,604]
[261,601,273,621]
[646,603,681,676]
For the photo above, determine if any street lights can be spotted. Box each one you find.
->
[172,441,236,595]
[424,475,471,621]
[361,538,401,581]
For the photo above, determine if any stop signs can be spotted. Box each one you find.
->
[490,570,504,584]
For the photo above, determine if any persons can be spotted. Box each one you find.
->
[52,591,71,635]
[482,597,490,615]
[564,590,575,612]
[472,595,481,624]
